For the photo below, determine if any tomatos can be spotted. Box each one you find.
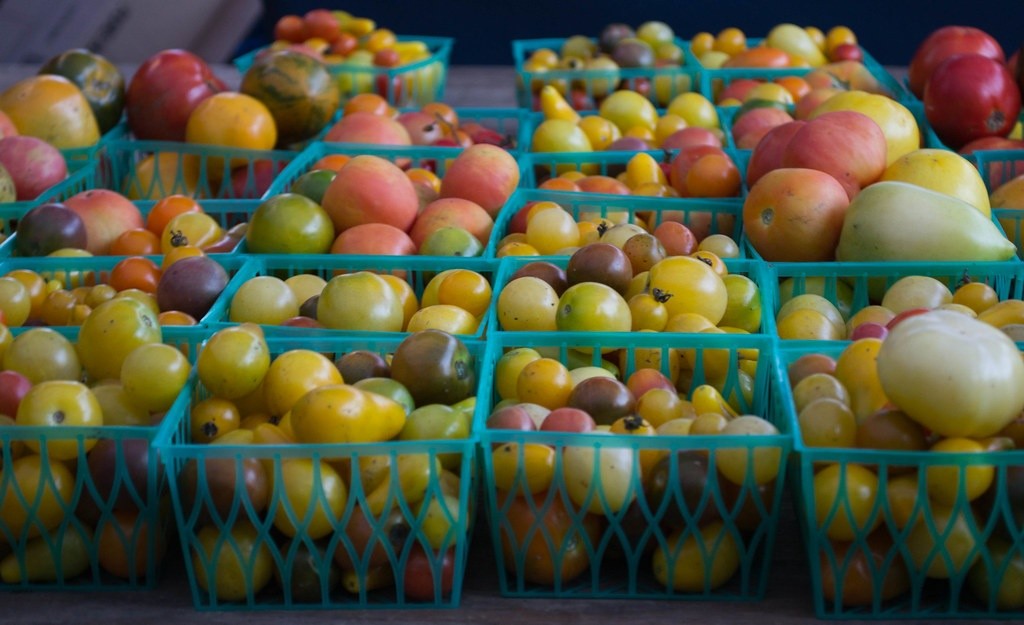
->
[0,7,1024,611]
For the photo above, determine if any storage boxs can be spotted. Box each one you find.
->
[0,35,1024,625]
